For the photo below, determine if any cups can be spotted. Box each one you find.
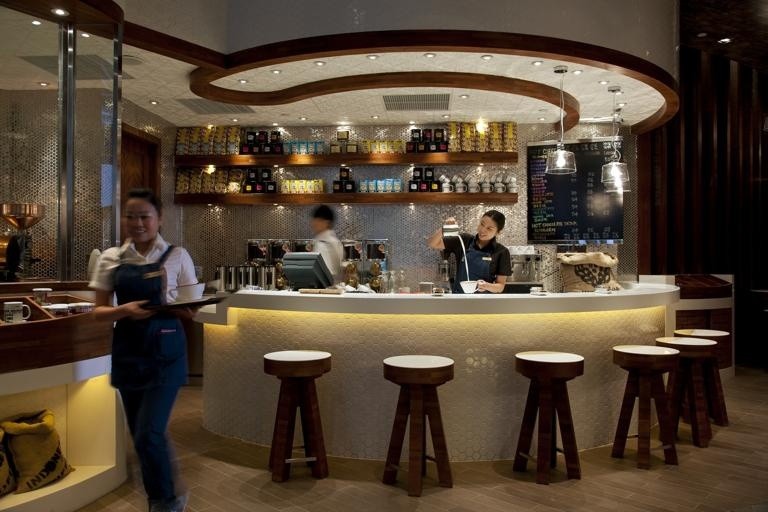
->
[441,183,519,196]
[458,280,481,294]
[441,219,460,239]
[3,300,32,323]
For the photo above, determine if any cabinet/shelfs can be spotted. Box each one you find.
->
[170,151,520,205]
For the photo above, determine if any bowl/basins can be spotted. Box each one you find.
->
[168,282,209,302]
[45,302,96,316]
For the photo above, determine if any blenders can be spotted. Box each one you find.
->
[434,249,454,293]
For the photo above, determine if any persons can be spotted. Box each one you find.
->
[89,186,202,512]
[428,210,513,293]
[304,204,344,289]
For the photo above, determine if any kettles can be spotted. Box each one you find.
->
[211,263,276,292]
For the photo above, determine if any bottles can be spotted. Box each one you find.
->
[379,269,402,295]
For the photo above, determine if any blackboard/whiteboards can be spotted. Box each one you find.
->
[526,135,625,245]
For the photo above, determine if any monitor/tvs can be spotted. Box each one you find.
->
[282,251,335,293]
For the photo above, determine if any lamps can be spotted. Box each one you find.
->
[545,66,631,195]
